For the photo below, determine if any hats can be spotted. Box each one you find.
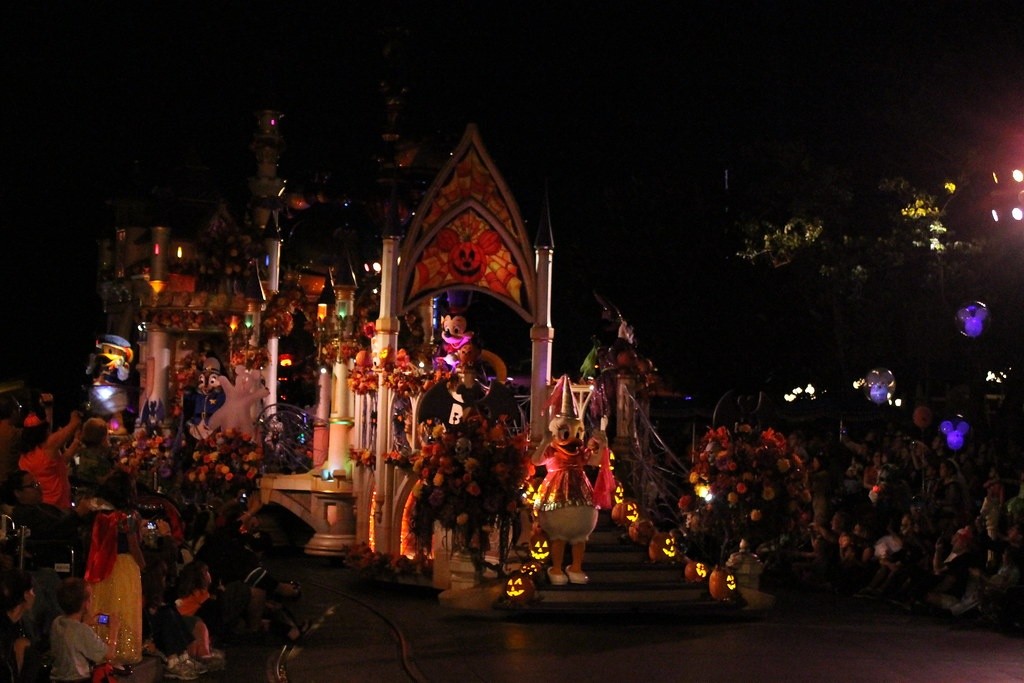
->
[23,411,48,427]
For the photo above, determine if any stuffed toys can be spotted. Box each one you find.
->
[187,356,270,442]
[440,306,484,404]
[86,335,134,434]
[531,413,610,586]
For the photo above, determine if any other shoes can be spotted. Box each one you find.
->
[887,599,911,614]
[164,661,199,679]
[179,653,208,674]
[865,590,884,600]
[193,650,225,671]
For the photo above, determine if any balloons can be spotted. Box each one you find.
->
[913,407,932,429]
[954,299,992,338]
[863,367,895,405]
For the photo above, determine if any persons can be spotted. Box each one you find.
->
[0,381,302,683]
[789,424,1024,614]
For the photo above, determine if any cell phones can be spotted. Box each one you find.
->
[98,614,109,624]
[147,521,159,530]
